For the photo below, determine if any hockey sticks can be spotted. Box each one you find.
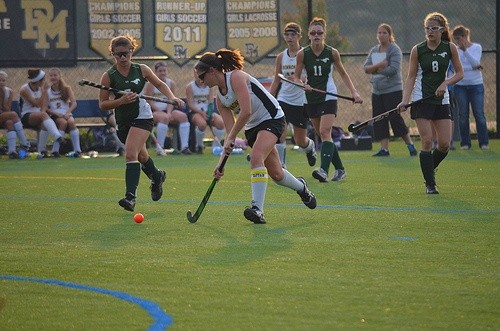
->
[187,142,234,224]
[78,78,178,107]
[277,73,355,101]
[347,90,447,132]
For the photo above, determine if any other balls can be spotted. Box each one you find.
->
[134,214,143,223]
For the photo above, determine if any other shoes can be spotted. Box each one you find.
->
[38,151,47,159]
[157,150,164,156]
[19,141,31,148]
[73,151,81,157]
[182,148,193,154]
[57,137,68,147]
[9,151,18,159]
[52,152,59,159]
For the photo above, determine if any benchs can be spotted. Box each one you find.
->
[0,97,219,141]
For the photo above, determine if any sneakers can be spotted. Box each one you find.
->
[306,141,317,167]
[119,192,136,212]
[296,177,317,210]
[427,184,439,194]
[332,168,346,181]
[373,149,390,157]
[312,168,328,182]
[150,170,166,201]
[243,205,267,225]
[407,143,418,156]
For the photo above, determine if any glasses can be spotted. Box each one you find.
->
[111,51,133,58]
[308,31,325,36]
[198,72,208,81]
[423,26,444,32]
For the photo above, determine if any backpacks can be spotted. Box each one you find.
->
[348,120,373,136]
[331,126,346,142]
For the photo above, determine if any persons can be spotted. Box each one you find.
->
[106,61,225,156]
[269,17,363,183]
[98,35,185,212]
[194,48,317,224]
[363,24,418,157]
[396,13,466,195]
[432,26,489,149]
[0,67,82,160]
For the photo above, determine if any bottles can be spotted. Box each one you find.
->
[66,151,79,157]
[212,135,223,156]
